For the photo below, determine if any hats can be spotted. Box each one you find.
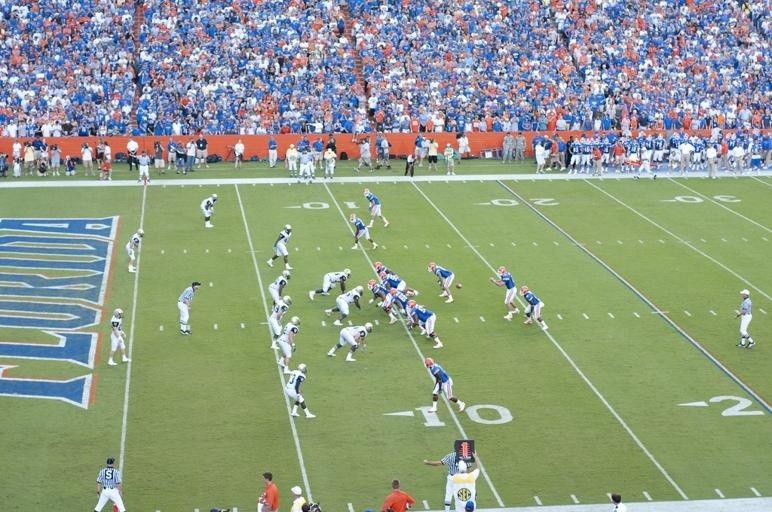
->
[739,289,750,295]
[290,485,303,496]
[191,282,201,286]
[458,459,467,472]
[105,457,115,464]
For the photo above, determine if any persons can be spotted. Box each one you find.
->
[94,458,126,512]
[385,480,415,512]
[200,193,218,228]
[108,308,132,365]
[125,229,144,273]
[610,493,626,512]
[451,450,480,512]
[177,282,202,336]
[424,444,473,512]
[734,289,756,349]
[290,486,307,512]
[258,472,279,512]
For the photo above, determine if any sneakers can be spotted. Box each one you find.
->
[309,290,314,301]
[325,309,330,316]
[437,291,454,304]
[458,401,466,413]
[269,342,293,376]
[284,262,294,270]
[501,307,533,325]
[367,222,389,229]
[735,340,756,349]
[180,329,192,335]
[321,292,330,296]
[350,242,379,252]
[291,412,300,417]
[127,266,137,273]
[419,327,444,350]
[107,356,135,366]
[205,223,214,228]
[541,325,548,331]
[266,260,276,269]
[428,407,437,412]
[333,321,343,326]
[327,351,336,357]
[305,414,316,419]
[387,288,420,325]
[345,358,356,362]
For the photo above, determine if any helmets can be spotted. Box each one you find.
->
[355,285,364,296]
[379,270,387,280]
[344,268,351,278]
[283,295,292,306]
[374,261,382,270]
[350,213,356,223]
[137,228,145,237]
[363,188,370,197]
[297,364,307,373]
[427,262,437,273]
[424,357,434,368]
[290,316,301,326]
[281,270,291,280]
[113,308,123,319]
[284,224,292,233]
[212,194,218,201]
[365,322,374,333]
[498,266,507,275]
[367,280,377,290]
[520,286,529,296]
